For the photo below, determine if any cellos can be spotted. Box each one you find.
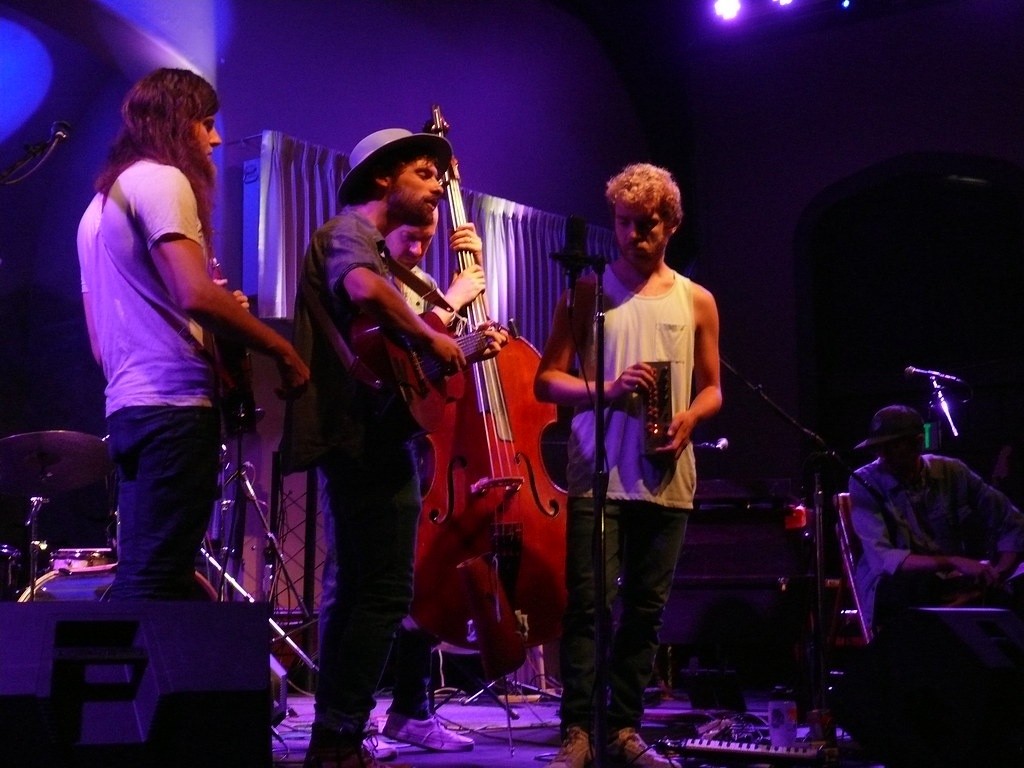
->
[411,100,575,755]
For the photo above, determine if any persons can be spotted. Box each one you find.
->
[533,162,723,768]
[849,405,1024,630]
[279,128,506,768]
[77,69,310,602]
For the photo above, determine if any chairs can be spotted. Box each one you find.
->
[830,490,878,649]
[425,639,546,760]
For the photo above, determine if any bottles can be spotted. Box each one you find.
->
[768,686,798,747]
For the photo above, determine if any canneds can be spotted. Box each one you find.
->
[809,708,835,745]
[768,700,796,747]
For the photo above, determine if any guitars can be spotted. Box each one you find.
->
[349,310,504,442]
[210,252,260,438]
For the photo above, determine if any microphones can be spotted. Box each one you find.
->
[216,461,250,501]
[564,214,589,320]
[715,438,729,451]
[904,366,964,384]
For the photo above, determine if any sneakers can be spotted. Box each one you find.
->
[382,712,474,750]
[304,746,392,768]
[361,735,397,761]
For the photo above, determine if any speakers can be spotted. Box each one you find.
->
[0,603,274,768]
[830,608,1024,768]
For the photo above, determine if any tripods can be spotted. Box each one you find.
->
[460,674,563,708]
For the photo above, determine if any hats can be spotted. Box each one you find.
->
[337,129,454,207]
[855,406,924,450]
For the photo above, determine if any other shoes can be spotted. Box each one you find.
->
[543,726,595,768]
[606,726,680,768]
